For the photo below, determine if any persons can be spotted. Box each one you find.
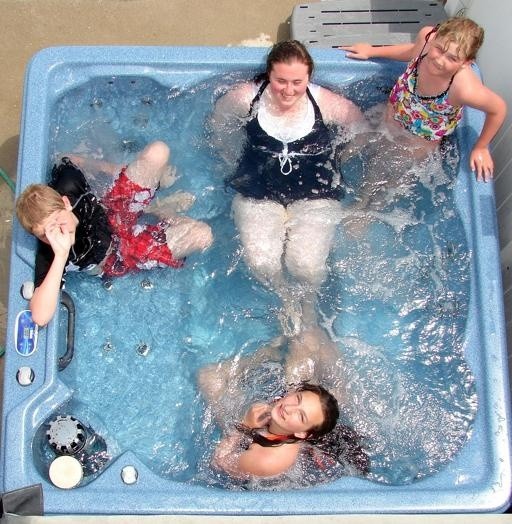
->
[256,38,333,112]
[15,163,120,327]
[338,10,507,182]
[194,327,370,492]
